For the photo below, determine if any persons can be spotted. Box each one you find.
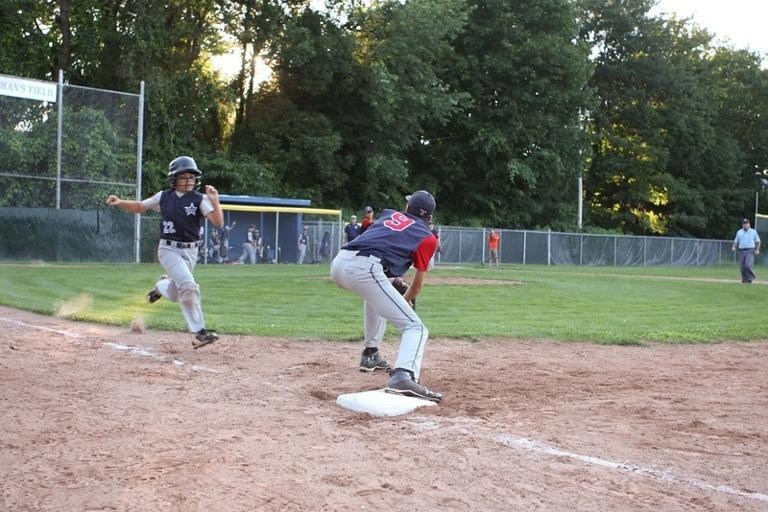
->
[105,155,225,350]
[199,221,330,264]
[429,223,440,270]
[344,214,360,243]
[331,189,443,403]
[488,228,500,267]
[732,218,761,283]
[358,206,375,235]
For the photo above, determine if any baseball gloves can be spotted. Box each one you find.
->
[391,278,416,310]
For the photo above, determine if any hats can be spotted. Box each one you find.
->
[741,218,748,223]
[364,206,373,213]
[404,190,436,215]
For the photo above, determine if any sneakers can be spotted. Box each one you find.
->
[359,351,390,372]
[145,275,168,304]
[384,371,442,403]
[190,329,219,349]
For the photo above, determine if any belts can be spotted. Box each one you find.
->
[166,240,197,248]
[355,251,370,257]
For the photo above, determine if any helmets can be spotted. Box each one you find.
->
[167,156,202,192]
